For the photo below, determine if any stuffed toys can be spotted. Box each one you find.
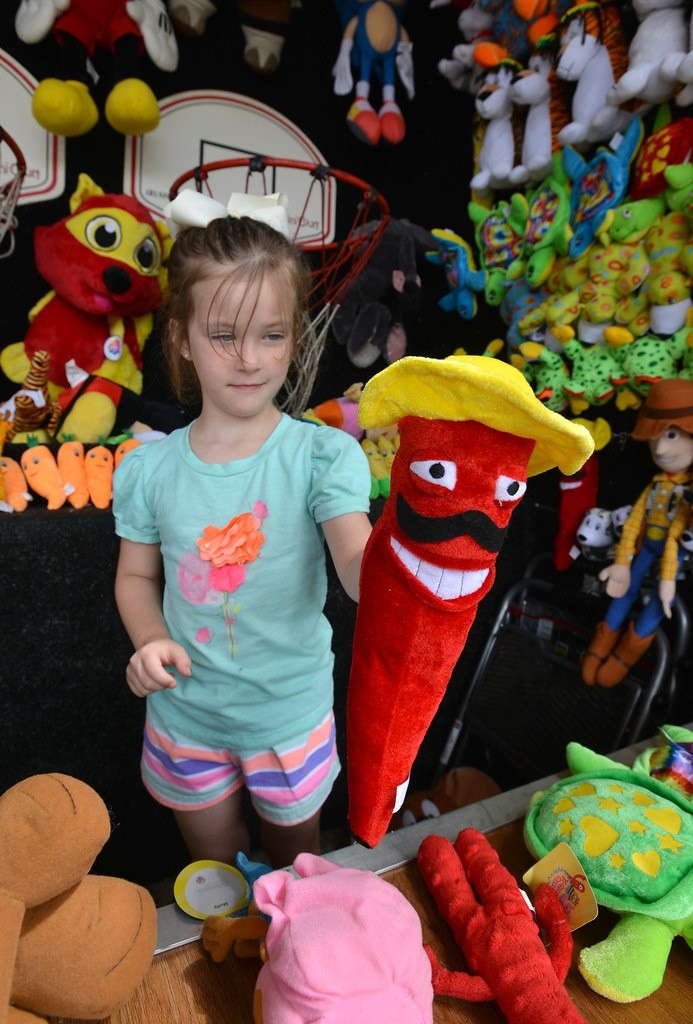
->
[0,1,693,1024]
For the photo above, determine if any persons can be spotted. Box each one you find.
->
[115,214,374,870]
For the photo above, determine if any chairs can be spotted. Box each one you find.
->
[433,555,690,776]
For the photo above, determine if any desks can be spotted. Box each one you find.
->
[47,723,693,1024]
